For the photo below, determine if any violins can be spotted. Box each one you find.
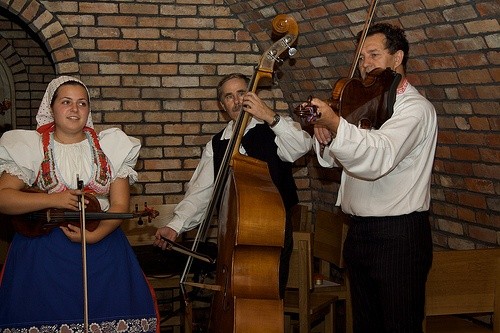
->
[12,193,160,238]
[296,67,401,130]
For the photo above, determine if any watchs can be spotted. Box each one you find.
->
[268,113,280,127]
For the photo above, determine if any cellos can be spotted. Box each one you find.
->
[181,16,299,333]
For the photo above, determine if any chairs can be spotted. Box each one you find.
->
[284,209,355,333]
[420,246,500,333]
[283,240,340,333]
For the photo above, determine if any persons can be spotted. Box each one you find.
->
[0,76,160,332]
[152,72,315,306]
[308,21,440,332]
